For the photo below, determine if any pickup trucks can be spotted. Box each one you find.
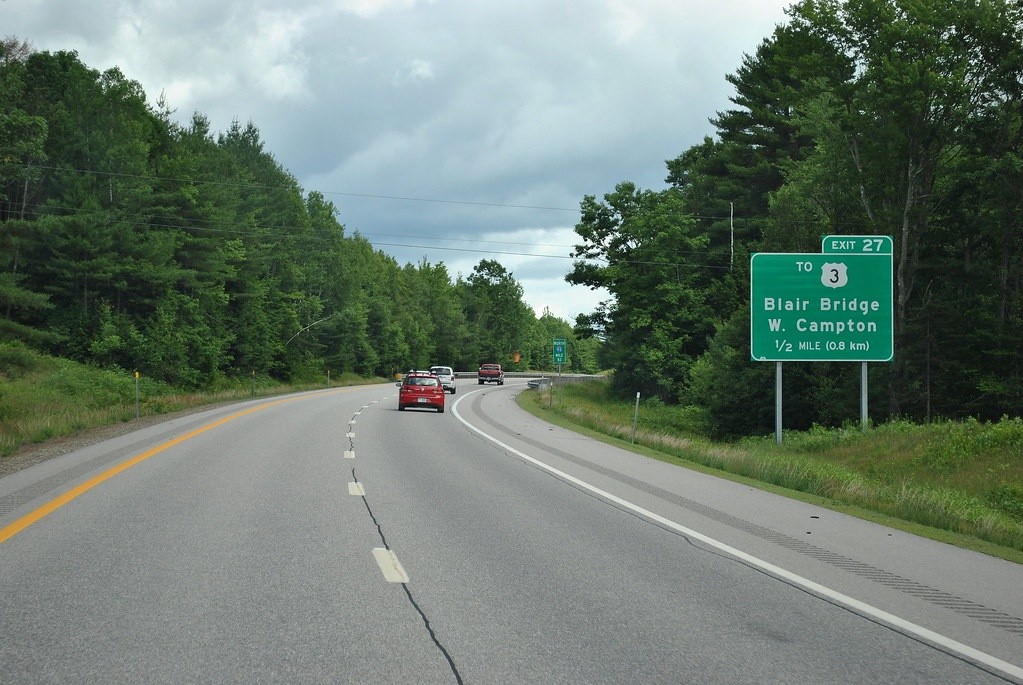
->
[431,365,457,395]
[476,363,504,386]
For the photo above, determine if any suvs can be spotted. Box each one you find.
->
[395,368,445,414]
